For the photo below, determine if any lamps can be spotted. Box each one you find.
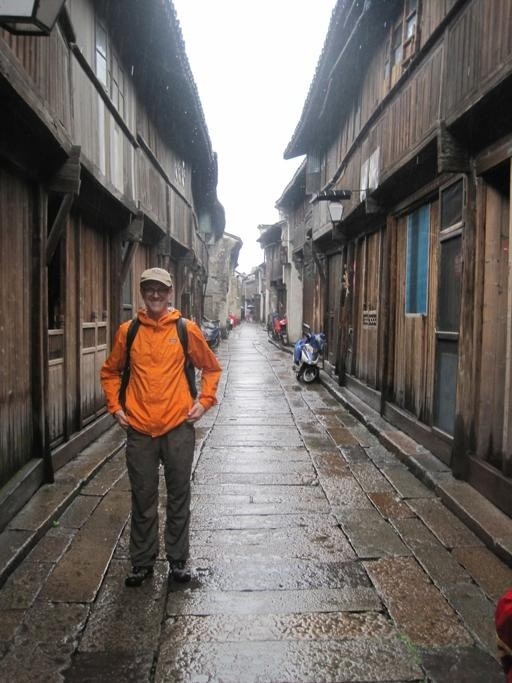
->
[326,200,355,240]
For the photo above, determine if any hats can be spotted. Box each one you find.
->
[140,267,173,288]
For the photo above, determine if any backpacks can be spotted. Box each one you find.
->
[118,316,198,411]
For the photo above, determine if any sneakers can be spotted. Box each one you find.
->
[124,565,153,588]
[168,559,192,582]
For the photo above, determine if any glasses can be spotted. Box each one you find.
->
[142,288,171,297]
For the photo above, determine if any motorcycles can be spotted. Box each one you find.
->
[199,314,221,352]
[291,321,327,383]
[272,315,290,345]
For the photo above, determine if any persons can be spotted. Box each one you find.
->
[100,267,222,586]
